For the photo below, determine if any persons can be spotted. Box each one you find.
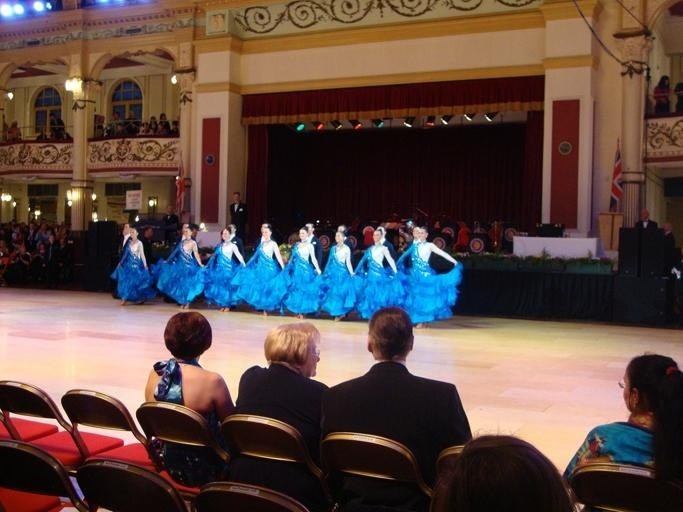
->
[674,83,683,113]
[2,113,181,144]
[162,204,179,241]
[1,218,76,290]
[230,192,247,246]
[563,354,683,512]
[662,222,675,245]
[233,320,331,511]
[144,310,237,491]
[109,222,462,330]
[317,306,473,511]
[653,76,673,114]
[635,209,658,228]
[430,433,578,511]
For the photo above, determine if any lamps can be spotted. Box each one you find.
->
[1,75,179,223]
[293,112,499,131]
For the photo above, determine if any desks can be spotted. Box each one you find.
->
[435,266,683,329]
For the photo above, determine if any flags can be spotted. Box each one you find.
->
[175,158,185,214]
[609,139,624,213]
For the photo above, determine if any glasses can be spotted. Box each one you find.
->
[617,379,629,391]
[311,348,320,362]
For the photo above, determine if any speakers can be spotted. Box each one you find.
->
[639,228,664,279]
[617,227,639,278]
[87,221,117,255]
